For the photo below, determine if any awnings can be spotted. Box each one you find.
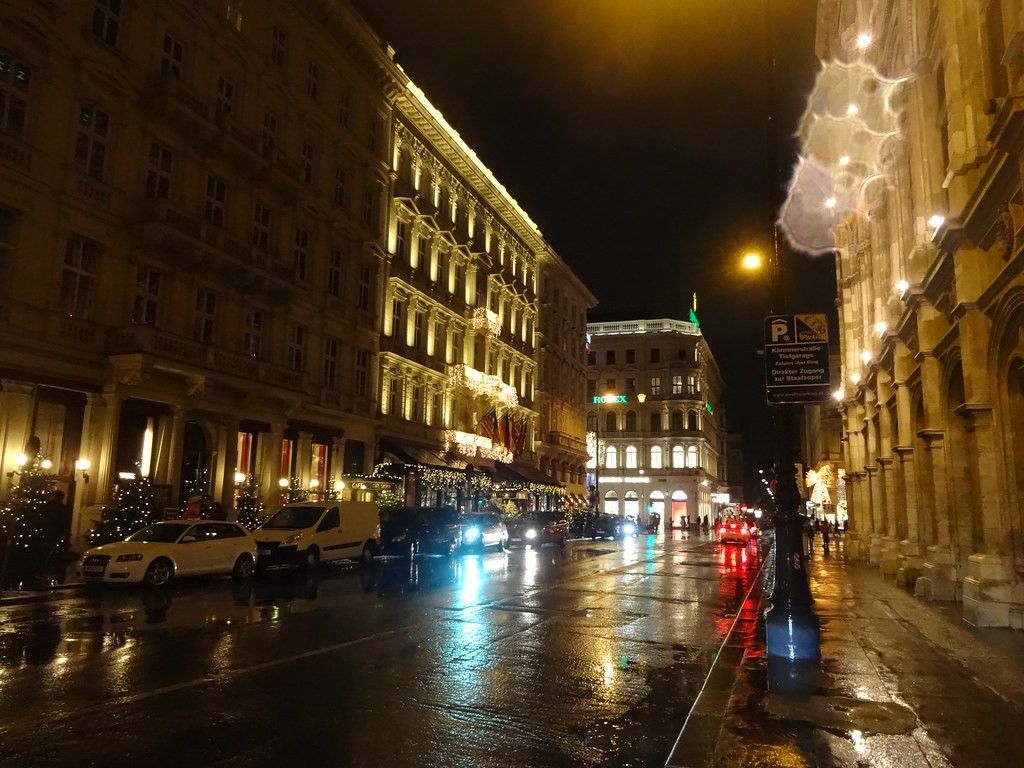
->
[380,436,479,475]
[496,460,561,487]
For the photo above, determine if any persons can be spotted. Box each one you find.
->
[832,520,842,536]
[694,516,701,531]
[648,512,674,531]
[814,518,820,533]
[687,515,690,530]
[680,516,686,530]
[807,525,815,548]
[636,515,641,525]
[820,522,830,548]
[703,515,708,532]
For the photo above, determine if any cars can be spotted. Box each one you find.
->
[720,519,751,545]
[79,519,259,588]
[743,518,760,538]
[591,515,640,541]
[506,512,570,550]
[461,512,509,555]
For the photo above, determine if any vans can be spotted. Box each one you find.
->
[381,506,462,562]
[253,501,381,569]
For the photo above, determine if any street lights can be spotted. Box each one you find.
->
[595,381,615,511]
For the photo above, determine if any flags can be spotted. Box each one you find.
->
[477,408,527,458]
